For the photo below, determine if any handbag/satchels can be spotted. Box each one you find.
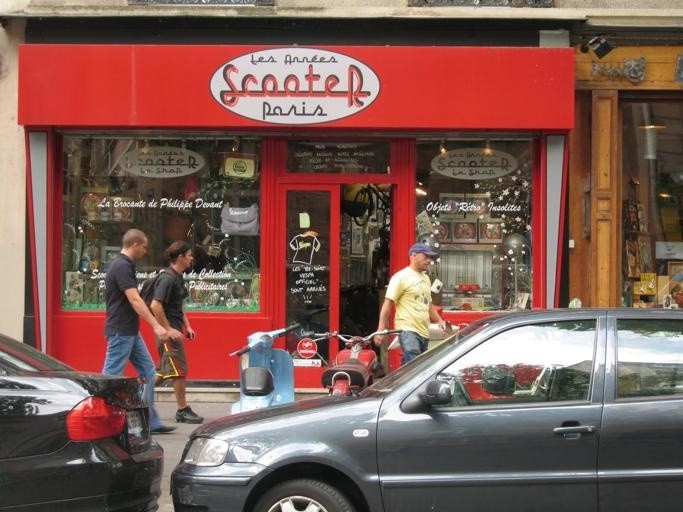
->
[220,202,259,236]
[185,218,222,257]
[139,277,156,305]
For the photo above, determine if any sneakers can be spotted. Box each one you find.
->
[175,406,203,423]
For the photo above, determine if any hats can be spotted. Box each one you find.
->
[408,242,438,256]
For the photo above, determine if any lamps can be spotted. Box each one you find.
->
[231,135,241,152]
[504,232,529,299]
[637,105,667,129]
[658,184,673,198]
[483,139,492,156]
[439,138,447,155]
[580,36,613,60]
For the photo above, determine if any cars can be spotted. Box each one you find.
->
[0,332,165,512]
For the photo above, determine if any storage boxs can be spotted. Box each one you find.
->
[428,193,504,244]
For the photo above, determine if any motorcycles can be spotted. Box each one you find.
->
[299,329,403,403]
[227,321,301,417]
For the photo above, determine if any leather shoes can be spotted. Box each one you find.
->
[153,425,175,432]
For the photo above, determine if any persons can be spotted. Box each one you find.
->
[97,227,178,432]
[373,243,451,367]
[143,239,205,424]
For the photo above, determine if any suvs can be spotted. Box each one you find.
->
[168,308,682,511]
